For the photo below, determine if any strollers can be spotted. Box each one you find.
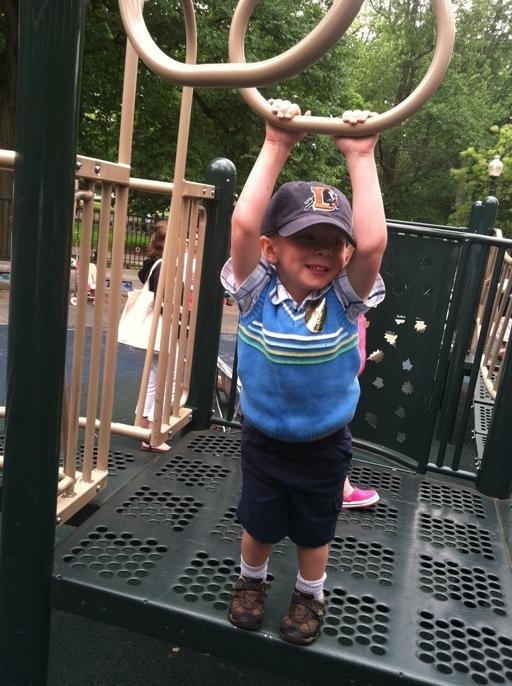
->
[215,355,243,431]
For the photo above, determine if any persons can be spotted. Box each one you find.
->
[134,223,184,454]
[181,227,199,312]
[220,97,389,645]
[339,242,379,511]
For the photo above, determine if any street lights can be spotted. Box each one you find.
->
[486,154,503,197]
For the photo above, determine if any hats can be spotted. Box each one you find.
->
[260,180,355,243]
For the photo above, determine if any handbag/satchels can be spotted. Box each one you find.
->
[117,286,163,354]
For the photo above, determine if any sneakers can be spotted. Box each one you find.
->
[280,589,324,645]
[141,441,170,453]
[230,578,267,631]
[343,486,379,508]
